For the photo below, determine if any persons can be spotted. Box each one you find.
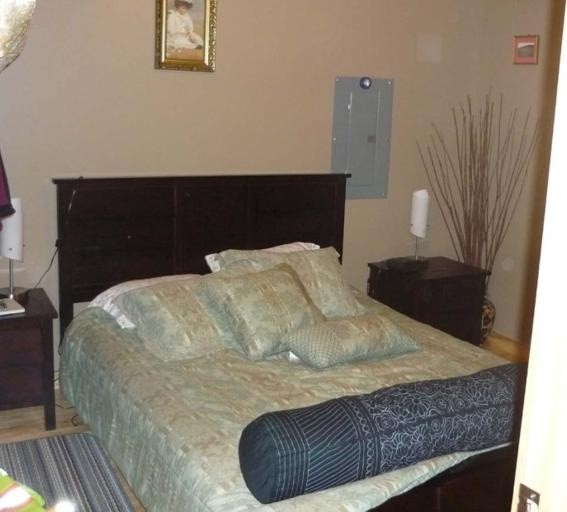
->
[166,0,205,50]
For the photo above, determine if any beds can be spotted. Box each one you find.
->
[49,173,523,510]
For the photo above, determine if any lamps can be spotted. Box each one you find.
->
[406,187,430,269]
[1,197,29,298]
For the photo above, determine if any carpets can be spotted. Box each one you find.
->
[1,428,135,511]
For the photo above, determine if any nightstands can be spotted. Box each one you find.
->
[365,254,490,349]
[0,286,61,432]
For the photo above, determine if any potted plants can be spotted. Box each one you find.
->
[414,83,544,343]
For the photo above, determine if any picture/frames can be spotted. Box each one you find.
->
[154,0,217,74]
[511,33,539,65]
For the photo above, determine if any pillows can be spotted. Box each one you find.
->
[240,359,528,504]
